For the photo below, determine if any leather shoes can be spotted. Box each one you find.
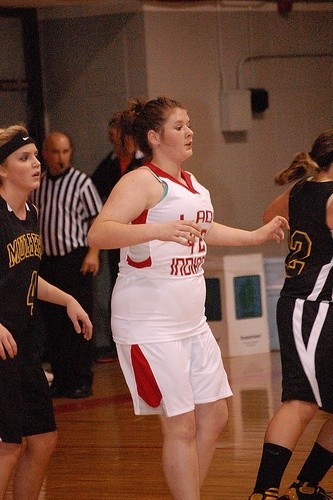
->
[67,383,92,397]
[49,383,69,397]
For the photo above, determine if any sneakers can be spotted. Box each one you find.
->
[285,479,333,500]
[250,487,280,500]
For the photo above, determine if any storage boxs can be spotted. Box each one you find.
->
[203,253,270,358]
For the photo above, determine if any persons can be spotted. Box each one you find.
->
[0,118,147,500]
[249,130,333,500]
[88,95,290,500]
